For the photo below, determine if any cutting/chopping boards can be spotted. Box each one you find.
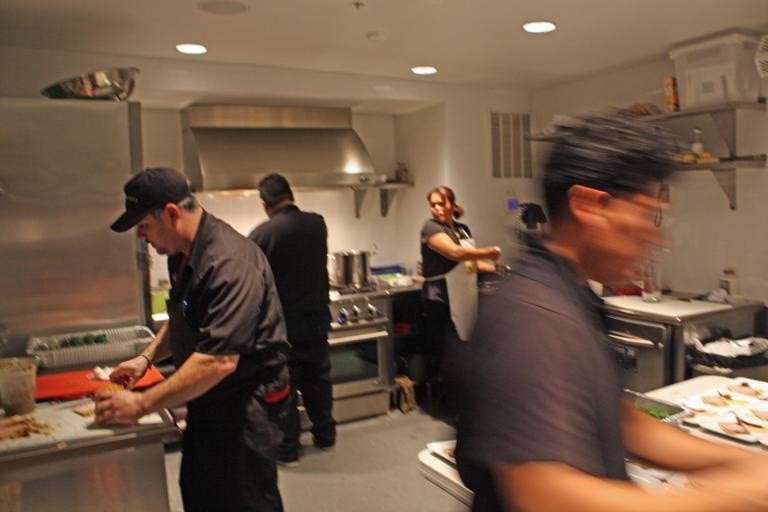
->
[0,392,173,455]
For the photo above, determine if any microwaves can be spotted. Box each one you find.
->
[327,331,392,425]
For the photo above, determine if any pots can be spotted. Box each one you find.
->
[329,249,372,291]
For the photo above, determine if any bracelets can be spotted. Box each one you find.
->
[137,354,152,368]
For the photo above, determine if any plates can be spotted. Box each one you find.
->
[673,377,768,450]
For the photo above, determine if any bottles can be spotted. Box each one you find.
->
[718,269,738,300]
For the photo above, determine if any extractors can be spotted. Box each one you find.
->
[179,99,388,194]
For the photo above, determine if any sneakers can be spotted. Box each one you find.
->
[277,437,336,468]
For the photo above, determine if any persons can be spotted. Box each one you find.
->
[92,166,290,512]
[245,173,335,464]
[419,186,507,408]
[456,111,768,512]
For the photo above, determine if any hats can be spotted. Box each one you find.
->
[108,166,188,232]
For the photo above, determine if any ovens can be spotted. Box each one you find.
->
[599,312,670,398]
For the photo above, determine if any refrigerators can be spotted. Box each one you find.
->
[0,100,154,356]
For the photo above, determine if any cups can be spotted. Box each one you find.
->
[638,252,664,303]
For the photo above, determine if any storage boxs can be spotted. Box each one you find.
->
[667,30,762,110]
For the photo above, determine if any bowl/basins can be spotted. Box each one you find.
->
[40,63,142,100]
